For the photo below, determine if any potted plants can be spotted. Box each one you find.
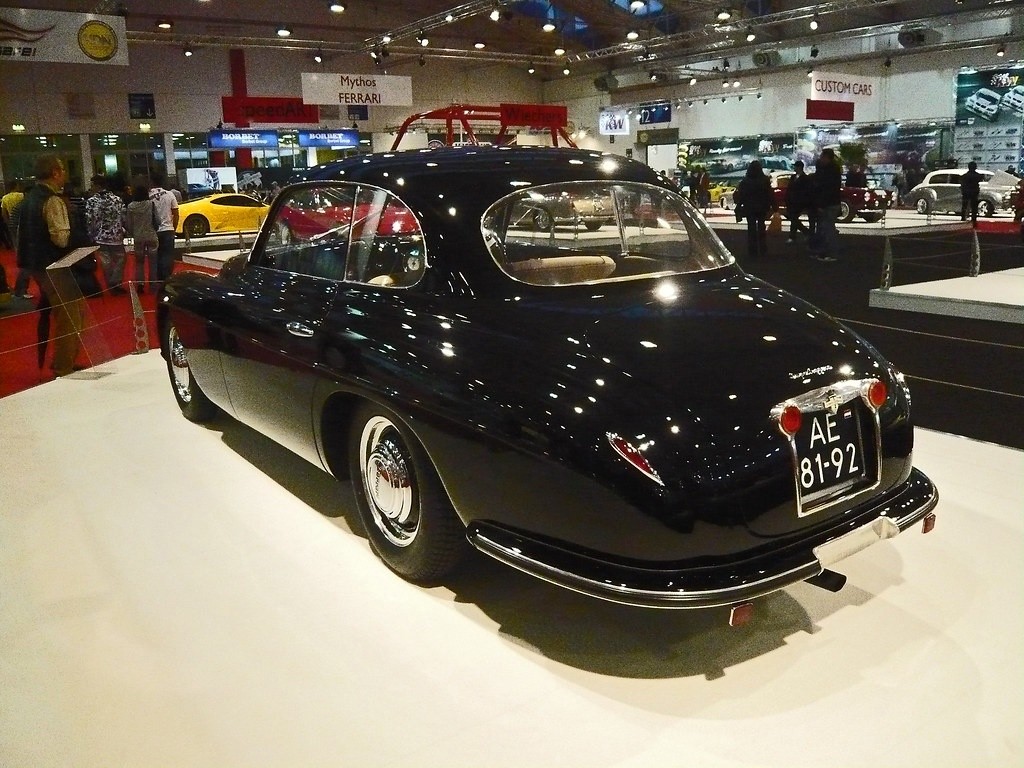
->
[838,141,868,171]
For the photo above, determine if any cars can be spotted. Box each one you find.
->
[1001,85,1024,113]
[706,182,736,202]
[690,153,793,173]
[764,172,896,223]
[272,189,421,247]
[187,183,214,194]
[490,191,658,232]
[904,168,1020,218]
[155,148,939,613]
[964,87,1002,122]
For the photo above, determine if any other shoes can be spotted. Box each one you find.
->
[109,283,126,295]
[786,238,794,243]
[137,283,144,294]
[20,294,34,300]
[817,255,836,261]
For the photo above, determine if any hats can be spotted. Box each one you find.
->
[792,161,804,166]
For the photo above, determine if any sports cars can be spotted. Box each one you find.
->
[175,193,272,238]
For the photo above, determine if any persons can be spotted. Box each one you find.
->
[661,149,1024,261]
[0,157,284,379]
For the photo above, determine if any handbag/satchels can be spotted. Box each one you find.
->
[152,202,159,232]
[769,211,782,232]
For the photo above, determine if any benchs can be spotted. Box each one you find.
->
[367,256,616,286]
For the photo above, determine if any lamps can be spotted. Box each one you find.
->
[155,0,1005,115]
